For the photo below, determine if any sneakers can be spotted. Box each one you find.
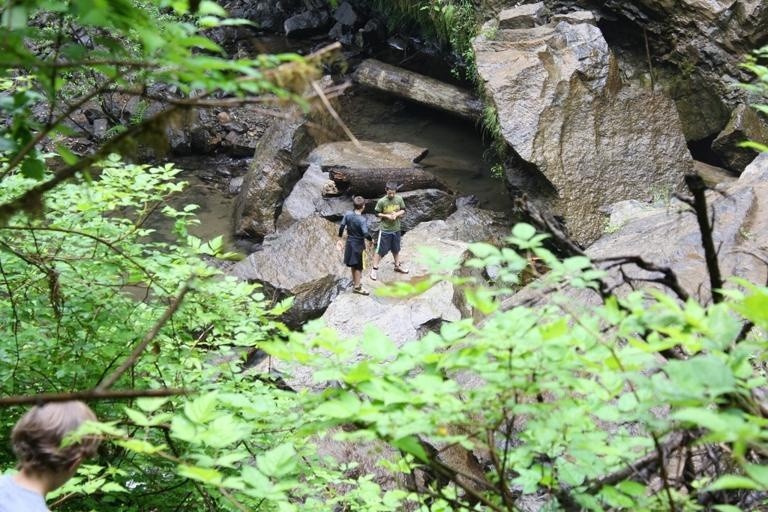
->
[352,263,409,295]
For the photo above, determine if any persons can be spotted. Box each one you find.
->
[370,181,409,281]
[0,399,103,512]
[338,196,374,295]
